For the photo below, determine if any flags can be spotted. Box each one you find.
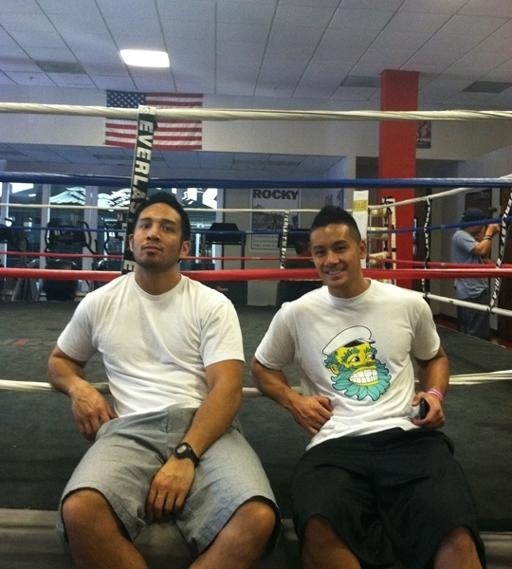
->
[104,89,204,151]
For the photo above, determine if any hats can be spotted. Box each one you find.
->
[458,208,482,229]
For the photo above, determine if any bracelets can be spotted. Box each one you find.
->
[427,387,445,402]
[483,235,493,241]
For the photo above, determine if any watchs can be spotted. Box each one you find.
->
[173,442,200,467]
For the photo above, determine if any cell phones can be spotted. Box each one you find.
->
[419,399,427,419]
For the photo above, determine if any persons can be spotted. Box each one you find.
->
[45,190,283,568]
[449,206,501,343]
[248,204,488,569]
[273,236,325,313]
